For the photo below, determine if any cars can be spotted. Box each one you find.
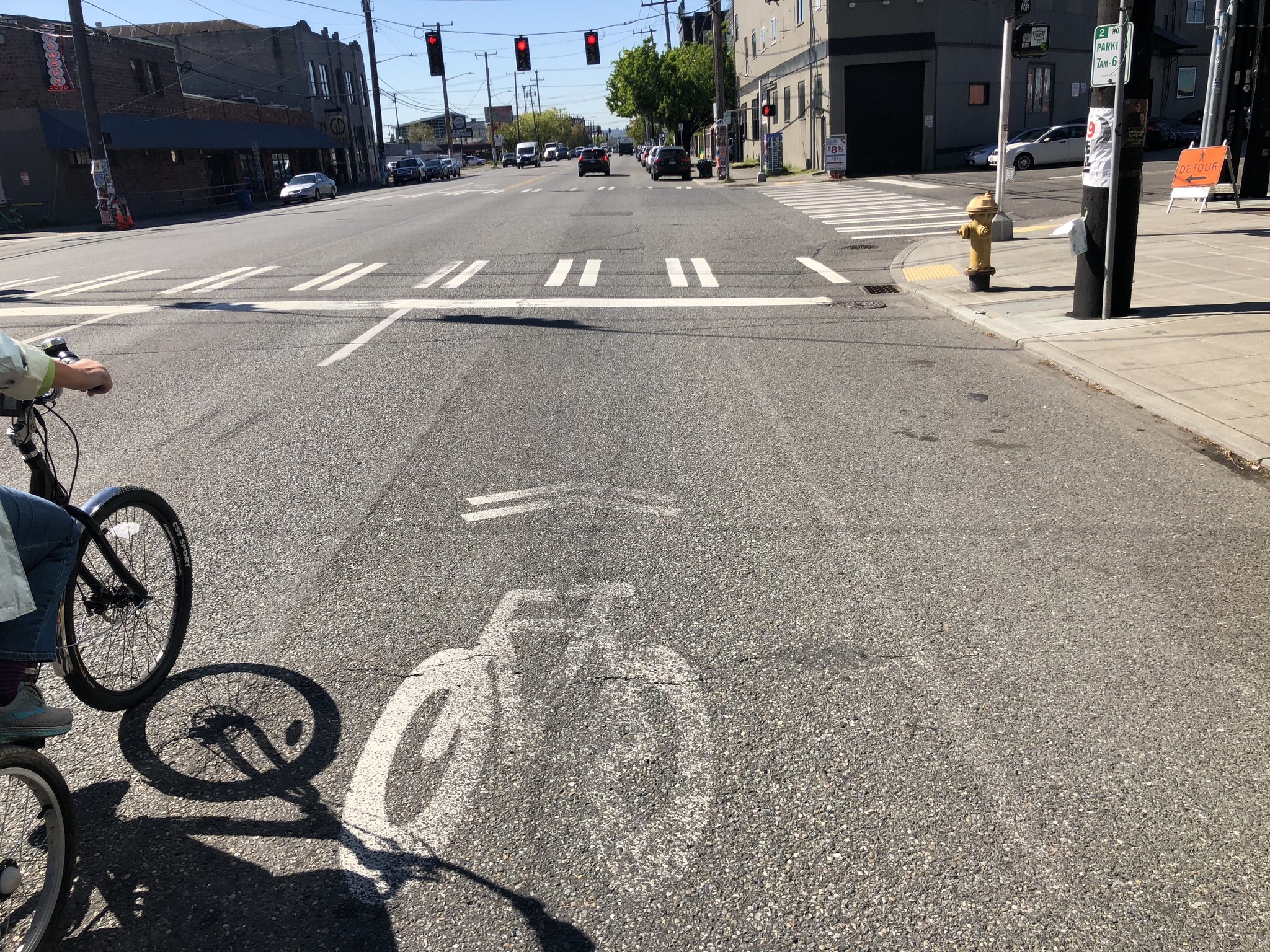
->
[280,172,338,205]
[387,161,398,174]
[466,156,486,166]
[1143,108,1205,153]
[961,118,1087,170]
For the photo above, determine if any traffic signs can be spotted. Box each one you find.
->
[824,135,847,171]
[1170,145,1228,188]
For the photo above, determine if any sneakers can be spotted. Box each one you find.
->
[0,690,73,744]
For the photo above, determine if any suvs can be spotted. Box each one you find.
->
[633,144,692,181]
[501,153,517,168]
[555,143,619,162]
[578,148,612,178]
[392,157,461,187]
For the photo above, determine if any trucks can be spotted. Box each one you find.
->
[544,140,563,162]
[618,137,634,156]
[514,142,541,169]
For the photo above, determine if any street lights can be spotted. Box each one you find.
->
[370,53,419,185]
[443,71,475,158]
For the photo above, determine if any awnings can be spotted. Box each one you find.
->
[39,108,344,153]
[463,143,492,150]
[1154,27,1198,49]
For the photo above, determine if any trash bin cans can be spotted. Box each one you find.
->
[698,159,714,178]
[235,190,253,212]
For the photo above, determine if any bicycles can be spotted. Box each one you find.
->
[0,338,194,952]
[0,199,27,230]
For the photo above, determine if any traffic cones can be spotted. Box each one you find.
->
[124,204,136,225]
[115,203,127,224]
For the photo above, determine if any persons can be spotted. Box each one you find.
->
[1123,101,1145,145]
[472,151,484,158]
[0,331,112,737]
[327,164,340,179]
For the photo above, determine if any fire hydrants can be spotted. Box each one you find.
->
[955,190,999,292]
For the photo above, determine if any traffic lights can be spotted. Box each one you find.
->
[424,32,441,77]
[762,105,776,116]
[514,37,531,72]
[584,31,600,65]
